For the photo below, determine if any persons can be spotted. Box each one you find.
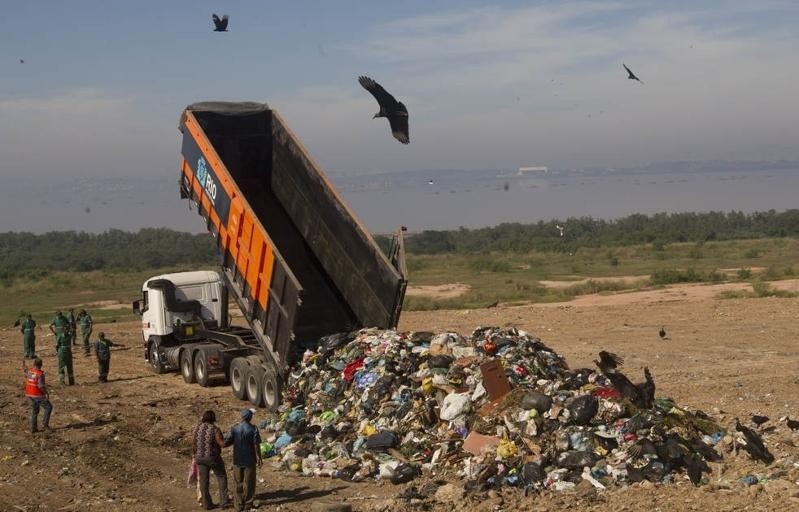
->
[66,306,78,348]
[20,355,54,434]
[93,330,125,384]
[55,323,75,387]
[215,408,264,512]
[48,309,71,355]
[191,409,234,511]
[75,308,95,358]
[20,313,36,360]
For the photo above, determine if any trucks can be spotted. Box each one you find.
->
[132,101,410,414]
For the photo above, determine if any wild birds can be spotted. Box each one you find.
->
[606,367,656,409]
[626,426,722,487]
[733,417,748,433]
[211,12,230,32]
[785,416,799,432]
[358,75,411,145]
[659,327,666,338]
[749,412,769,431]
[622,61,643,83]
[592,350,624,376]
